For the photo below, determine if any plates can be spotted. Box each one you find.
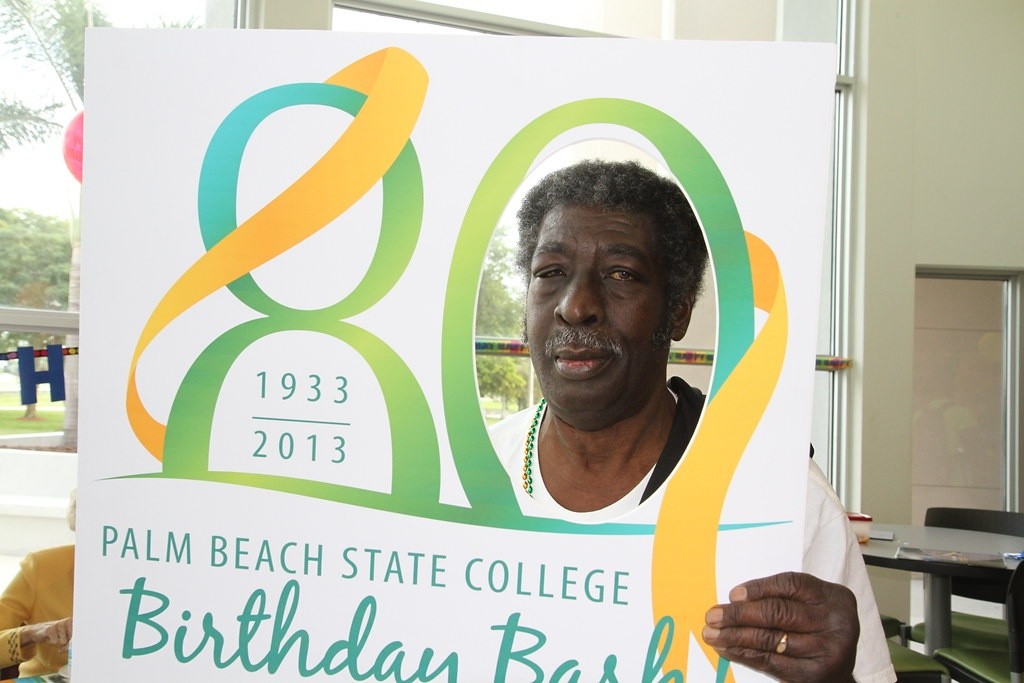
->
[58,663,71,680]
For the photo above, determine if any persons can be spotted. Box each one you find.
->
[486,160,898,683]
[0,489,77,678]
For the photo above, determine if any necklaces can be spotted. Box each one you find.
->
[522,399,546,496]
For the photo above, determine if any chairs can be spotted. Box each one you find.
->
[879,507,1024,683]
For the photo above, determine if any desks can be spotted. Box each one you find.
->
[849,519,1024,683]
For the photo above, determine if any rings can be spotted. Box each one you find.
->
[776,633,788,653]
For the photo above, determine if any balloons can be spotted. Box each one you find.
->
[63,112,84,185]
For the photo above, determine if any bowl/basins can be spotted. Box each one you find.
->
[846,512,873,543]
